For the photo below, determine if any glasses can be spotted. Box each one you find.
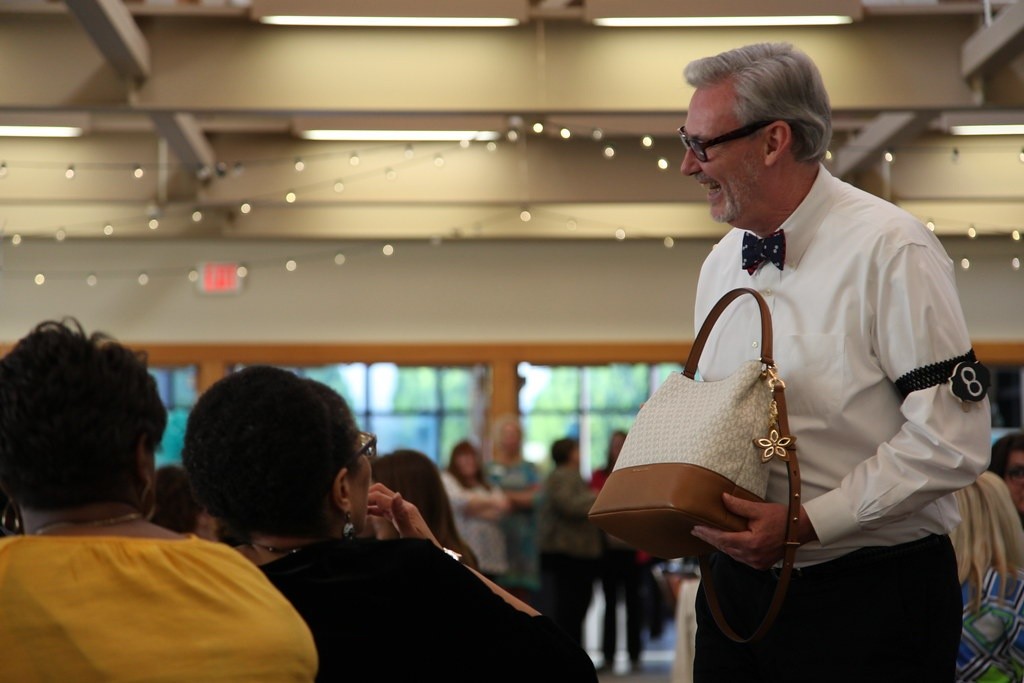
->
[677,121,774,163]
[343,431,377,468]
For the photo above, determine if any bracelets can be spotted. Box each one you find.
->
[443,547,463,563]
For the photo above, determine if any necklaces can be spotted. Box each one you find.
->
[33,509,142,534]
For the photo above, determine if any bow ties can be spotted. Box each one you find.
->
[741,228,785,277]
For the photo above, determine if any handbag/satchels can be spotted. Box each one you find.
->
[587,287,801,645]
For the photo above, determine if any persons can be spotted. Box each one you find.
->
[368,449,481,569]
[439,441,510,587]
[948,471,1024,683]
[984,432,1024,530]
[586,429,652,674]
[0,313,319,683]
[538,437,600,650]
[682,43,993,683]
[182,366,598,683]
[484,415,550,601]
[150,466,211,538]
